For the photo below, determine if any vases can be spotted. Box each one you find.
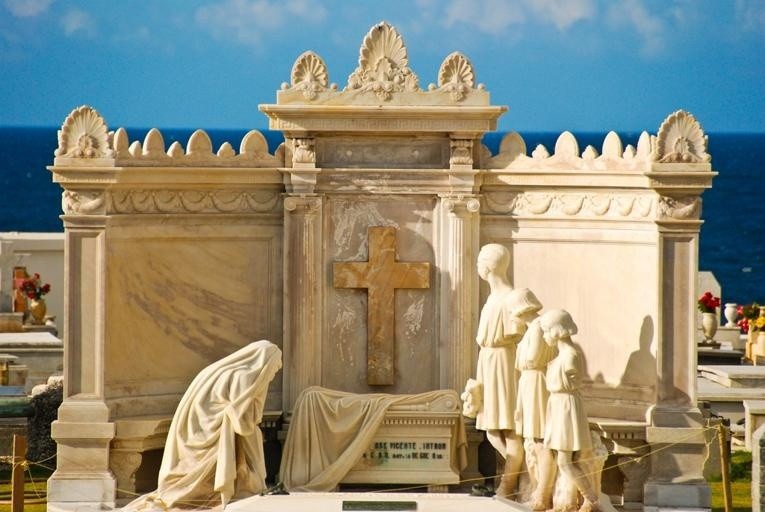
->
[29,299,46,325]
[745,332,765,359]
[702,312,717,343]
[724,303,739,327]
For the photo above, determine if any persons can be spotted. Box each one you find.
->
[121,338,282,512]
[528,308,604,512]
[465,243,530,504]
[507,287,582,512]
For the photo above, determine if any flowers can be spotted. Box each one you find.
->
[698,292,720,313]
[736,303,765,332]
[17,273,50,300]
